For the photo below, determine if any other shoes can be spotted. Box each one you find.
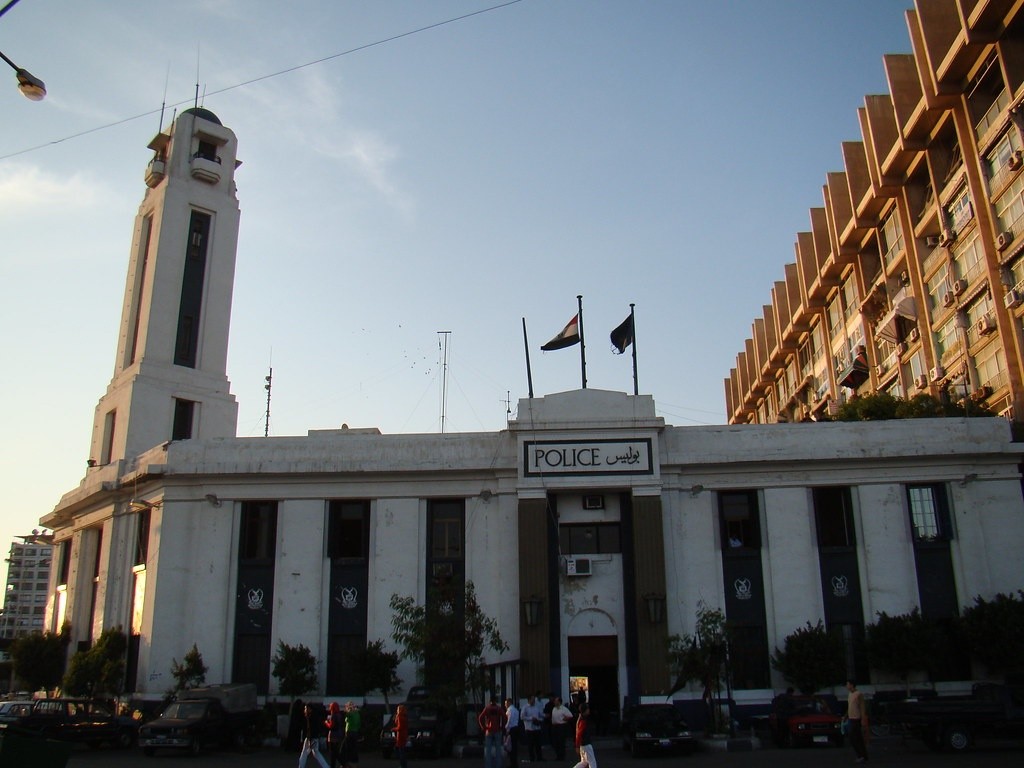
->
[855,756,865,764]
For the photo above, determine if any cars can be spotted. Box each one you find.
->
[380,700,445,758]
[769,692,845,744]
[1,697,134,754]
[620,703,694,756]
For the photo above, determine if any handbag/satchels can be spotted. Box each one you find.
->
[503,735,512,753]
[841,718,849,736]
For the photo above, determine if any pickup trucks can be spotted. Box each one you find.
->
[138,699,280,760]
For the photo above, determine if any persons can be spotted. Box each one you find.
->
[391,705,410,768]
[480,697,519,768]
[842,679,869,763]
[772,687,795,749]
[299,704,330,768]
[324,701,361,768]
[573,703,597,768]
[520,691,573,760]
[577,687,586,704]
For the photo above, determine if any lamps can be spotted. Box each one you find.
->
[204,492,221,508]
[129,499,161,511]
[87,460,107,467]
[689,483,703,498]
[646,592,664,623]
[523,595,543,627]
[960,472,978,488]
[479,488,491,505]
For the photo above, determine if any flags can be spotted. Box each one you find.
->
[610,314,632,354]
[541,313,580,351]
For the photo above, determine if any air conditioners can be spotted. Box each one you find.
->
[803,393,819,414]
[896,343,906,356]
[900,270,909,282]
[930,367,943,382]
[926,229,953,247]
[1003,290,1020,309]
[943,279,965,307]
[957,386,989,409]
[995,232,1011,252]
[584,496,604,510]
[910,328,919,342]
[977,317,991,335]
[565,558,593,577]
[875,364,885,377]
[1007,151,1022,171]
[914,375,927,389]
